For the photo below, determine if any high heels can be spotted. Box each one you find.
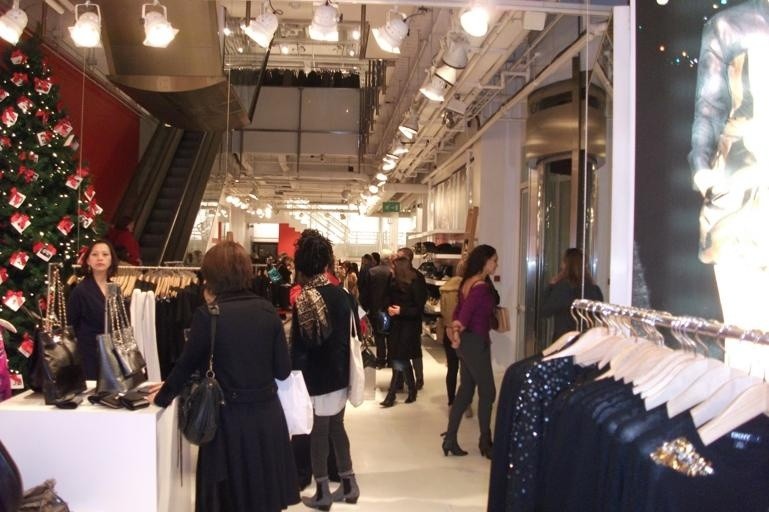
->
[478,440,494,460]
[440,432,468,456]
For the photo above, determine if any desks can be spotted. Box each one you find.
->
[1,381,197,512]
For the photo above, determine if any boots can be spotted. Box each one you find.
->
[332,472,360,503]
[430,265,452,279]
[302,477,332,511]
[380,370,401,407]
[403,366,417,403]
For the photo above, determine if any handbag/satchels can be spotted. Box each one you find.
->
[495,305,511,332]
[347,336,366,405]
[177,377,225,444]
[359,313,374,339]
[95,326,148,394]
[275,369,314,436]
[22,320,86,405]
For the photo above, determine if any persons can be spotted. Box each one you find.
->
[439,258,473,418]
[442,245,501,461]
[691,0,768,381]
[70,238,132,381]
[537,247,602,346]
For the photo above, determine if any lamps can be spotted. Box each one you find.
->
[367,0,505,197]
[232,179,355,222]
[0,0,412,58]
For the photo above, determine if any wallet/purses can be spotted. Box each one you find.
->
[139,383,162,395]
[88,391,150,410]
[57,394,83,409]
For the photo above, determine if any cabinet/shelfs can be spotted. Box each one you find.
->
[409,232,479,347]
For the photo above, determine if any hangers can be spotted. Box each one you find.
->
[63,262,204,298]
[545,295,767,447]
